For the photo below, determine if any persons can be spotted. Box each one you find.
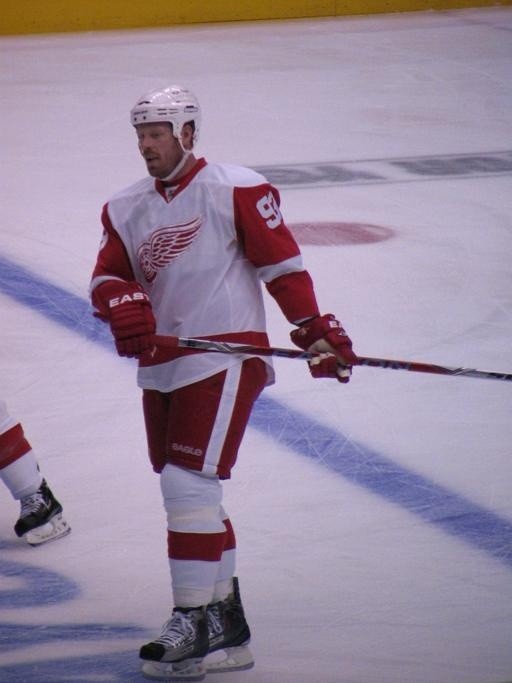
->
[87,85,357,679]
[0,394,72,551]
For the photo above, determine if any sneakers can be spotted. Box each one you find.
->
[15,478,62,537]
[139,592,251,663]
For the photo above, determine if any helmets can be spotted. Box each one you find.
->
[131,85,200,138]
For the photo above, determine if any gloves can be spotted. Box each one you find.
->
[290,314,356,383]
[92,279,156,358]
